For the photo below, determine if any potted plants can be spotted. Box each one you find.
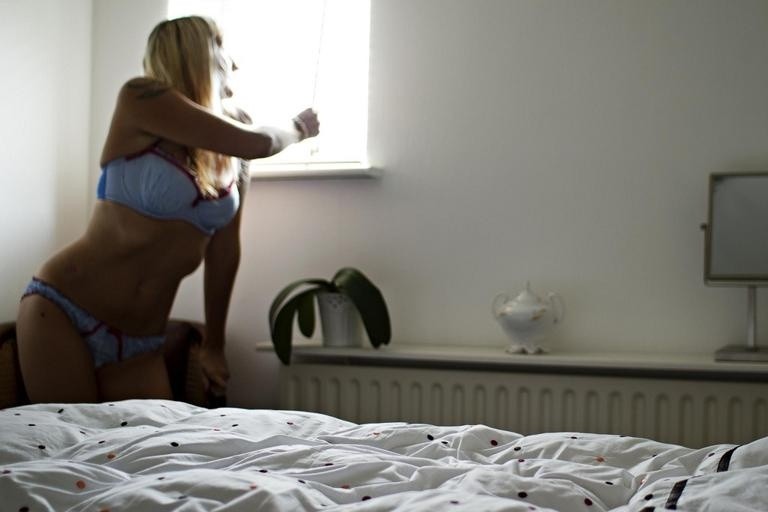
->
[265,261,393,368]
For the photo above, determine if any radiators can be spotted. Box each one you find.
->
[256,341,767,456]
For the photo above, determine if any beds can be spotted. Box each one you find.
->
[0,391,767,512]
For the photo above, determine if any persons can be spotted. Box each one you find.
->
[17,17,321,404]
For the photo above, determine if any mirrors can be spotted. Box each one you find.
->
[695,171,766,367]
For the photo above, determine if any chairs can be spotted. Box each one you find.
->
[1,312,224,412]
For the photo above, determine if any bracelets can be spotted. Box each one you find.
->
[293,117,309,140]
